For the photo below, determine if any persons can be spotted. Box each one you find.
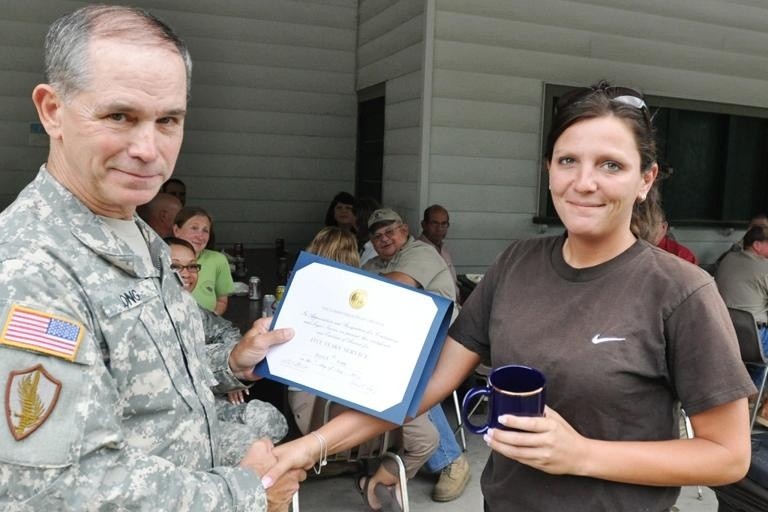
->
[636,204,768,429]
[147,180,472,500]
[0,4,308,512]
[261,82,759,512]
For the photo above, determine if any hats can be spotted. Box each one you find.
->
[368,208,404,226]
[744,227,768,244]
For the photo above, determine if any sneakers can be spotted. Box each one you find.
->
[756,401,768,426]
[430,452,469,503]
[748,394,768,409]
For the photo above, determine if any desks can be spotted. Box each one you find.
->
[214,267,311,373]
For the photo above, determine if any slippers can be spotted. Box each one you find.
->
[374,482,402,511]
[352,465,379,510]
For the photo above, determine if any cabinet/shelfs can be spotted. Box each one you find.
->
[533,81,768,235]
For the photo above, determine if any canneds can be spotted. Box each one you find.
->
[236,257,245,279]
[280,258,289,274]
[234,242,242,258]
[275,237,285,257]
[262,294,277,318]
[249,277,261,299]
[274,284,285,304]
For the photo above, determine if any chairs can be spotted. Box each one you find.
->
[720,306,768,437]
[292,390,420,512]
[451,389,468,451]
[454,357,493,438]
[678,408,704,501]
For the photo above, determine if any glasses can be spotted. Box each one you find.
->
[371,223,403,240]
[171,263,201,273]
[430,220,450,226]
[553,87,653,132]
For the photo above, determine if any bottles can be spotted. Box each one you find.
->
[461,365,545,435]
